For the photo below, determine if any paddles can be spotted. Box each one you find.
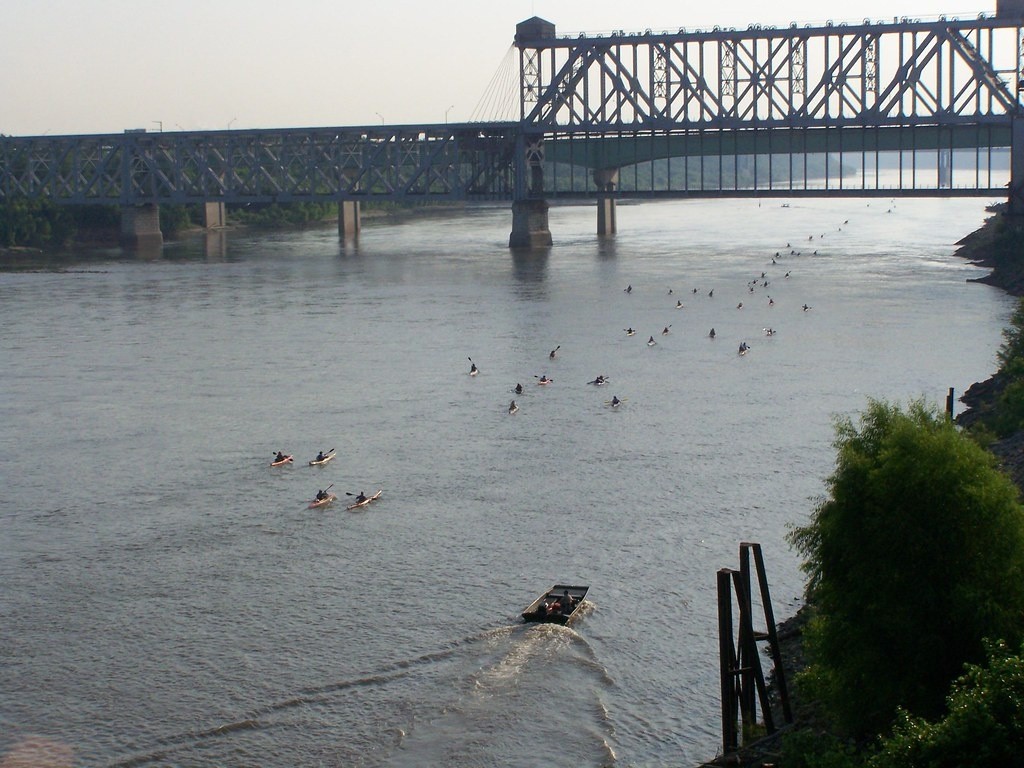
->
[666,325,672,328]
[623,328,635,332]
[273,452,278,456]
[322,484,334,491]
[468,356,474,364]
[346,492,371,499]
[324,449,335,455]
[587,377,611,384]
[553,346,561,351]
[604,398,628,403]
[534,376,554,381]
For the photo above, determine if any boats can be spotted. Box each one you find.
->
[595,379,605,386]
[309,452,336,466]
[469,369,477,376]
[626,332,636,336]
[308,492,336,510]
[270,454,294,467]
[739,348,748,356]
[515,389,522,394]
[521,583,590,629]
[509,405,519,415]
[539,379,551,385]
[347,489,382,511]
[611,401,620,408]
[648,340,655,347]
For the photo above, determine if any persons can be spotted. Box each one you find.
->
[356,492,367,503]
[316,451,326,461]
[560,590,573,614]
[471,350,621,409]
[317,491,326,500]
[627,220,849,353]
[276,451,288,460]
